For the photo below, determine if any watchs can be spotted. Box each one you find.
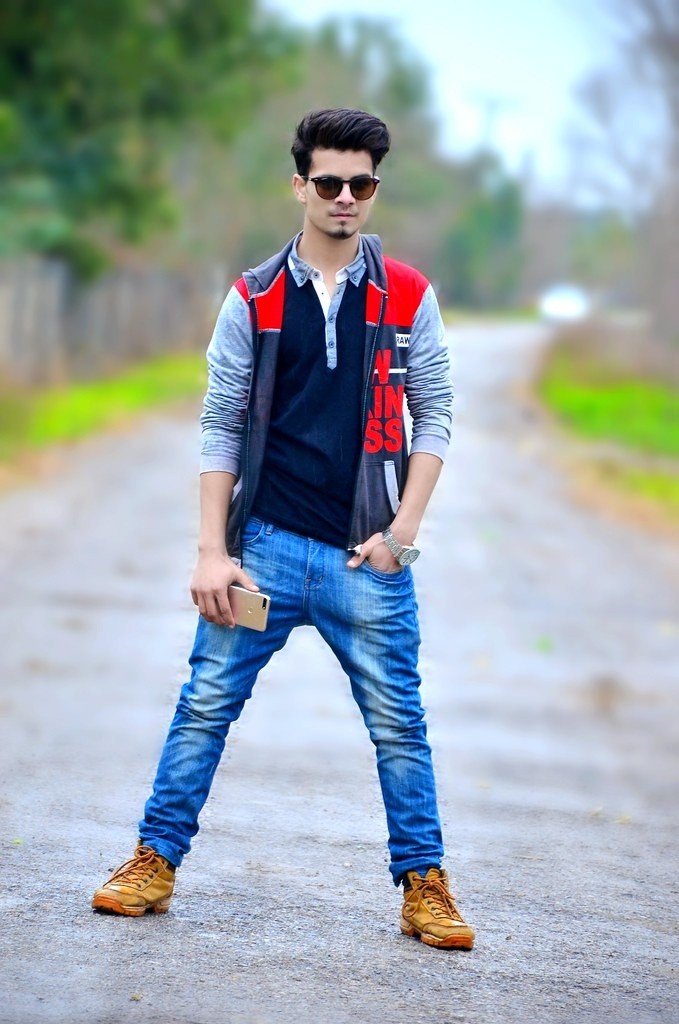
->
[382,531,422,568]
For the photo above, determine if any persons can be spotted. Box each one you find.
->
[91,109,474,955]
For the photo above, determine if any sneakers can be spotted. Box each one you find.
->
[400,869,474,951]
[92,841,175,915]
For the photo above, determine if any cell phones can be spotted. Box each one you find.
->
[227,584,270,632]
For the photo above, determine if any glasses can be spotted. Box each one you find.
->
[302,176,380,200]
